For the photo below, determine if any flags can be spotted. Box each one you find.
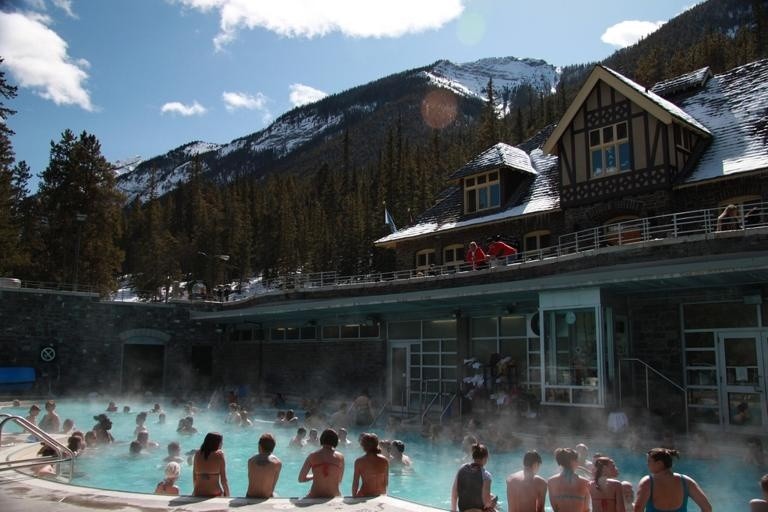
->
[385,209,397,233]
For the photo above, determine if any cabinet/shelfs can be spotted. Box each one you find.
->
[460,356,522,414]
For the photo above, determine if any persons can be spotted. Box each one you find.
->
[593,232,610,247]
[183,272,230,301]
[466,241,490,270]
[285,278,295,289]
[427,263,438,275]
[745,206,761,227]
[13,368,767,512]
[716,205,742,230]
[488,237,517,264]
